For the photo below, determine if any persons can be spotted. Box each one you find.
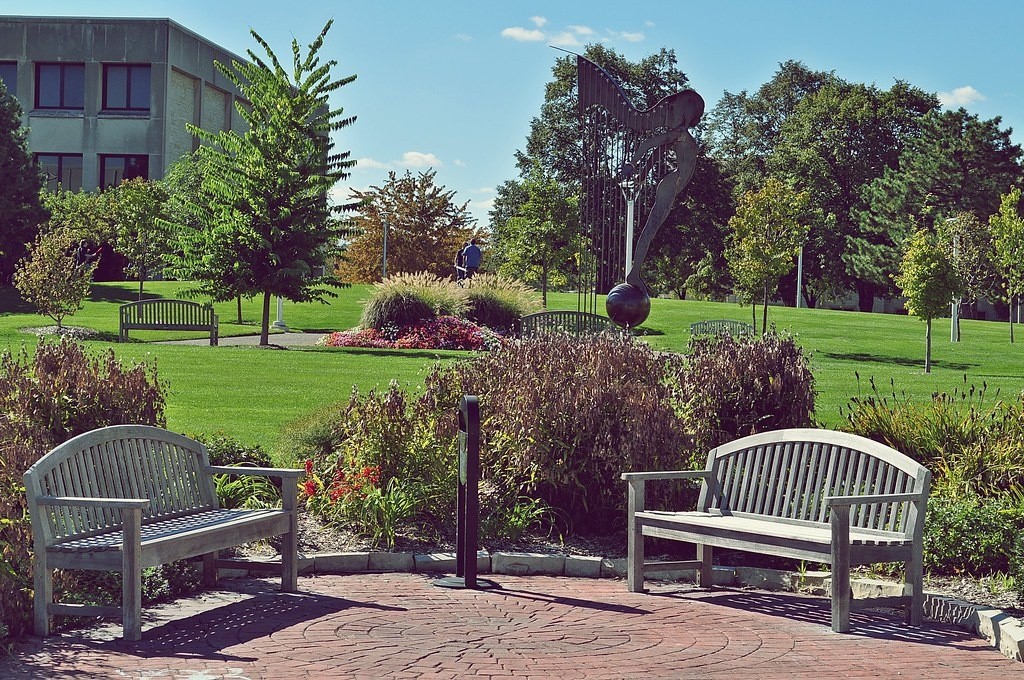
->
[75,240,97,284]
[620,89,704,291]
[454,238,482,284]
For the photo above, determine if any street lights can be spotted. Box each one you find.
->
[380,210,391,278]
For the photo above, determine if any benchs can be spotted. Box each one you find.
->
[22,424,305,643]
[621,427,933,633]
[520,311,615,340]
[119,298,219,346]
[691,320,753,338]
